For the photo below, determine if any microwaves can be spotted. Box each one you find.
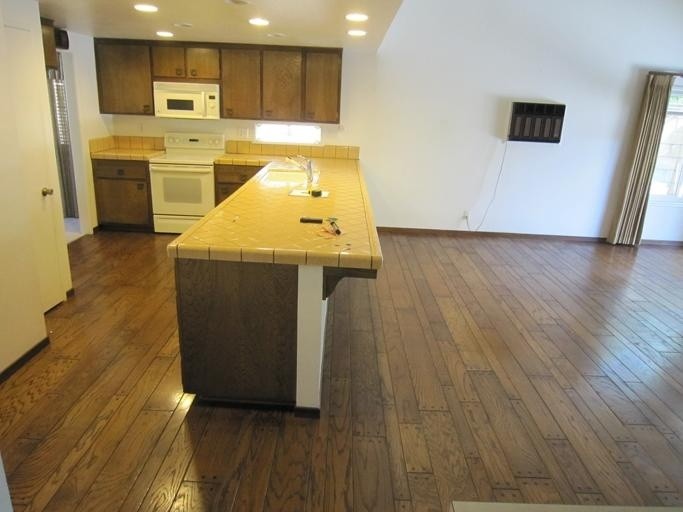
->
[152,80,220,120]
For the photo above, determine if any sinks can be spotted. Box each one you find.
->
[258,166,321,186]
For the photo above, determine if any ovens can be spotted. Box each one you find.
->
[148,162,214,234]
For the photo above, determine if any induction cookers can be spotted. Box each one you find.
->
[148,150,226,166]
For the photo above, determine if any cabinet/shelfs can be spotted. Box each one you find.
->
[261,50,302,121]
[214,164,261,206]
[149,44,220,80]
[39,16,57,70]
[94,162,150,227]
[96,42,154,115]
[219,48,262,119]
[304,47,343,123]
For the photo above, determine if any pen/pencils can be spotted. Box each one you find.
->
[330,219,341,235]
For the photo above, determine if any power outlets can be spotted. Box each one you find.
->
[464,210,470,220]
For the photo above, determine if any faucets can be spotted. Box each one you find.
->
[285,153,314,184]
